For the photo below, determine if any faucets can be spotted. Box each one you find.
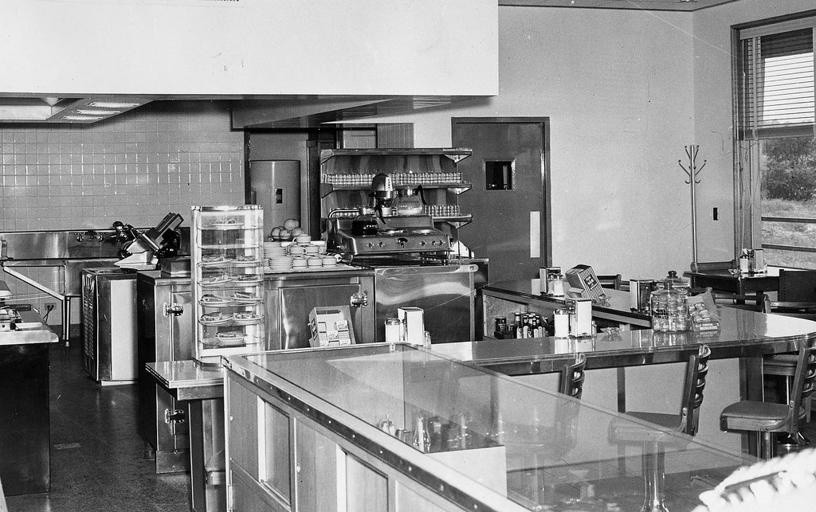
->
[96,233,106,243]
[74,232,85,242]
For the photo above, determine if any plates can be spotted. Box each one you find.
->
[263,241,293,272]
[197,223,262,347]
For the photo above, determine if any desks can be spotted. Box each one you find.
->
[683,271,780,314]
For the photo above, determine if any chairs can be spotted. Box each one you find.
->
[501,352,599,508]
[608,344,711,512]
[765,269,816,320]
[691,259,737,308]
[718,331,816,457]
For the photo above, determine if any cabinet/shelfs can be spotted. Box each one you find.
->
[319,146,473,262]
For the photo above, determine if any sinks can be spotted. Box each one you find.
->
[64,257,119,299]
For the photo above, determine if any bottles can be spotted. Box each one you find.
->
[649,270,690,333]
[726,260,738,270]
[493,316,506,332]
[553,308,597,340]
[383,316,432,349]
[376,404,479,452]
[739,250,755,273]
[512,310,542,340]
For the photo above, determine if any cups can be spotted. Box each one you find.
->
[685,301,709,321]
[285,236,337,267]
[329,204,460,217]
[327,170,463,185]
